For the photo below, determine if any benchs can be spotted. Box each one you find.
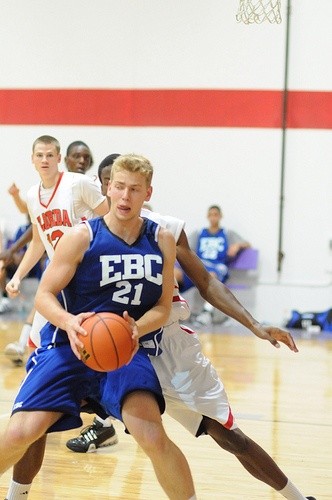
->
[224,249,258,310]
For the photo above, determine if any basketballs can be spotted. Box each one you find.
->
[74,313,136,373]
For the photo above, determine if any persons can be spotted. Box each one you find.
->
[0,153,198,500]
[4,154,318,500]
[0,136,252,452]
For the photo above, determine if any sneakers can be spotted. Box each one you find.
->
[3,342,24,367]
[65,417,119,455]
[0,293,13,315]
